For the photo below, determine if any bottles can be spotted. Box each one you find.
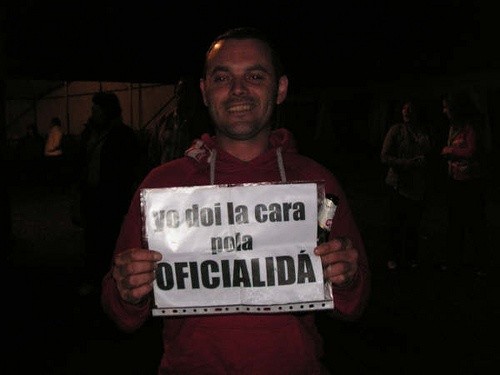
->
[317,193,339,284]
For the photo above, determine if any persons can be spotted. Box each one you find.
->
[69,90,143,296]
[381,101,433,270]
[438,97,476,194]
[18,124,42,149]
[100,26,371,375]
[44,117,65,158]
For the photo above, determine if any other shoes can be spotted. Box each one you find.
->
[388,260,397,269]
[407,260,420,268]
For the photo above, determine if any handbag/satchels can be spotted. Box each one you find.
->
[444,126,479,183]
